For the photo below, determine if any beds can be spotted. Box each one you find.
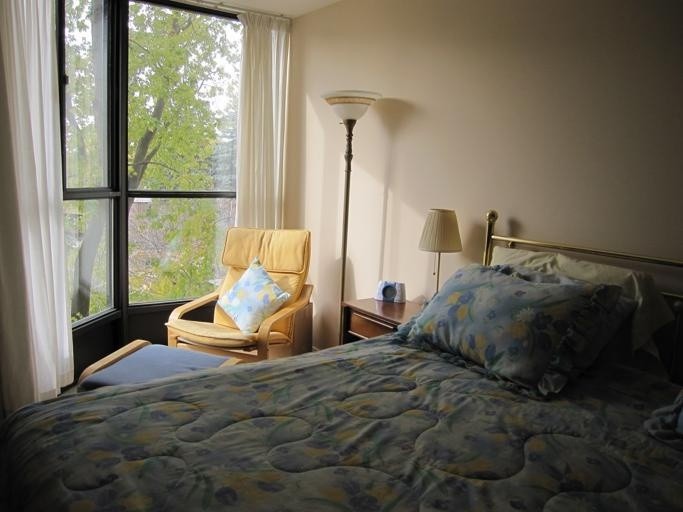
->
[0,210,683,512]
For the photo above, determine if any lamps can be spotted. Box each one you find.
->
[419,208,463,293]
[321,89,382,345]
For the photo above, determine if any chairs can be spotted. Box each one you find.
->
[164,227,314,363]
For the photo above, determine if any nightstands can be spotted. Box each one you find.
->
[340,297,423,346]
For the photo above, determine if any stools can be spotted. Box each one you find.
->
[76,339,244,395]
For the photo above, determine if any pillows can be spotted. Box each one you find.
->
[217,257,293,334]
[408,260,623,402]
[600,295,638,356]
[639,388,683,451]
[491,245,668,365]
